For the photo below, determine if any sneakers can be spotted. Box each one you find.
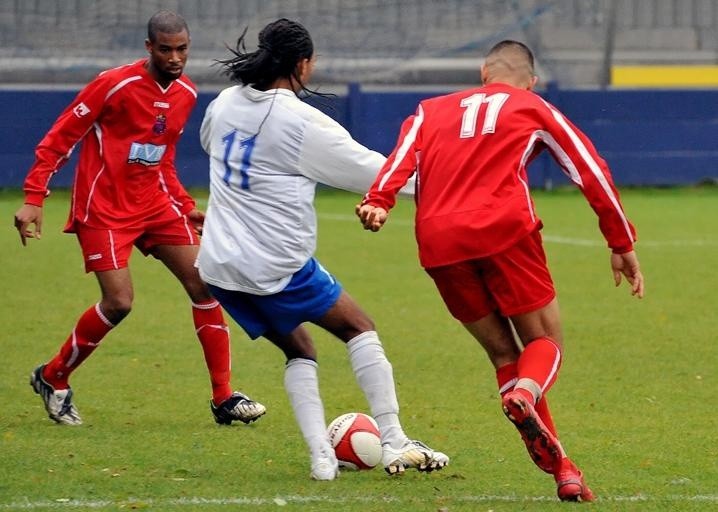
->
[28,360,83,427]
[307,448,344,484]
[206,389,270,427]
[378,437,453,475]
[498,388,568,476]
[554,473,594,502]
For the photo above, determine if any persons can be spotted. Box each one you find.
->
[193,16,449,481]
[352,40,645,506]
[14,10,265,430]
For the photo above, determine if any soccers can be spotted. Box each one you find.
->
[326,412,382,471]
